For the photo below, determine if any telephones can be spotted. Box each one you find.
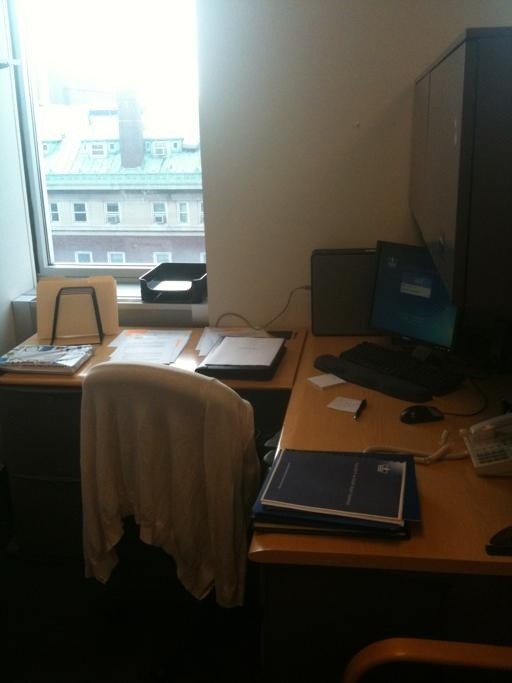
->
[456,413,512,476]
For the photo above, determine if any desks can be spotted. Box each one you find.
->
[1,324,309,561]
[247,330,512,665]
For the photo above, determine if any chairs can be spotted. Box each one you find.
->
[344,639,512,681]
[79,361,260,616]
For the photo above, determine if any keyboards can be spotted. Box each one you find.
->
[338,339,465,391]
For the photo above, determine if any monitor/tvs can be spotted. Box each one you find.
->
[365,240,461,362]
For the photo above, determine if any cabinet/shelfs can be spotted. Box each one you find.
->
[408,25,512,380]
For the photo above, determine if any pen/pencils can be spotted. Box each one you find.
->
[353,400,366,421]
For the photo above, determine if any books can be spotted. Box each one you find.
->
[250,450,419,538]
[1,345,94,374]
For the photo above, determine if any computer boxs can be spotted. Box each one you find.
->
[311,248,374,336]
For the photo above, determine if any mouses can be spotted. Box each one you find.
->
[400,404,445,424]
